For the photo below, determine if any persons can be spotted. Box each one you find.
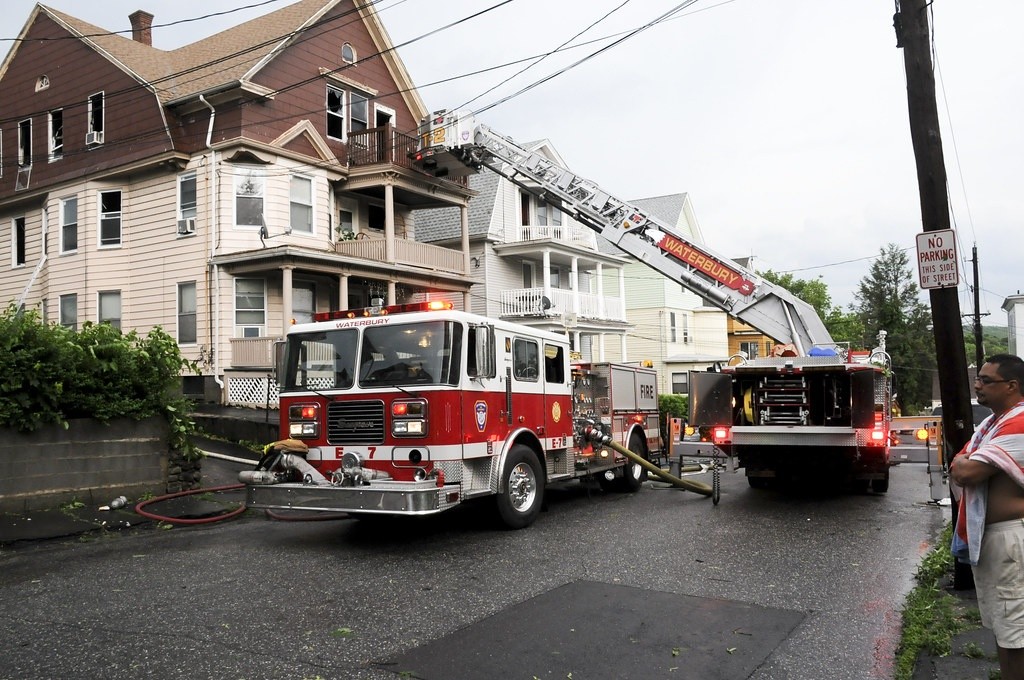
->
[949,353,1024,680]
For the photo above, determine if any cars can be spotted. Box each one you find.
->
[929,399,995,434]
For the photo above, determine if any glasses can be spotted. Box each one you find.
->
[973,375,1013,385]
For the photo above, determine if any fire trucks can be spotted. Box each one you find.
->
[238,295,667,530]
[406,104,949,509]
